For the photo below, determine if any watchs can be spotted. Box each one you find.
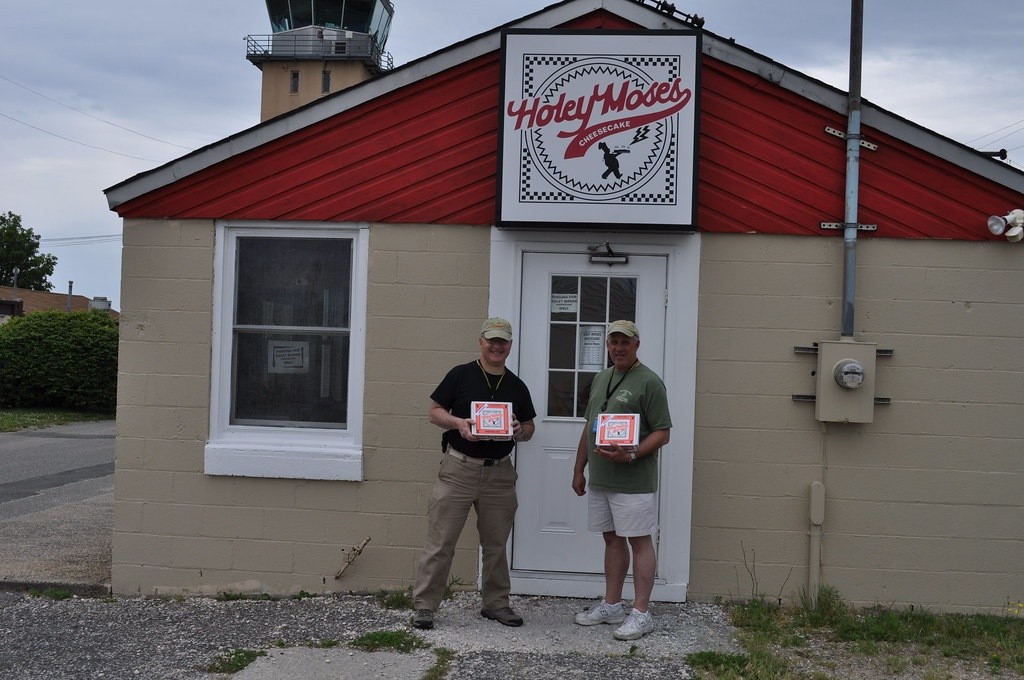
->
[628,453,639,465]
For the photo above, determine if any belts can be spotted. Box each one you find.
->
[449,449,512,465]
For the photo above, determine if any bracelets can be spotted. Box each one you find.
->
[513,427,524,442]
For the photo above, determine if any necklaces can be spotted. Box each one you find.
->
[476,359,506,400]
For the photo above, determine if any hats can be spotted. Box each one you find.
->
[607,320,639,338]
[480,316,512,341]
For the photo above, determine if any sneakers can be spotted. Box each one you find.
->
[413,609,434,628]
[481,606,523,626]
[613,608,654,640]
[575,596,625,625]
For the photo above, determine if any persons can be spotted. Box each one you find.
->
[572,321,673,640]
[412,318,536,629]
